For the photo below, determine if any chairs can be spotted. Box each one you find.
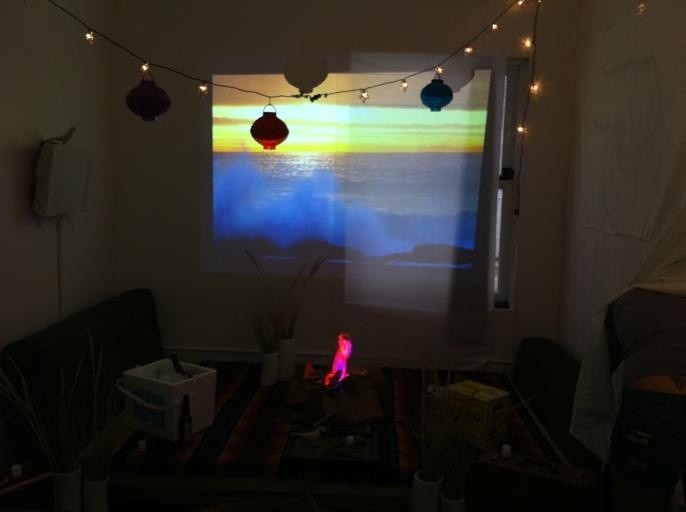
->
[511,335,604,473]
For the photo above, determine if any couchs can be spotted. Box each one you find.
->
[1,288,170,481]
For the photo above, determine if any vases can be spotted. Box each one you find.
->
[277,337,297,382]
[81,474,110,512]
[409,469,443,512]
[439,492,466,512]
[260,351,277,387]
[52,464,81,512]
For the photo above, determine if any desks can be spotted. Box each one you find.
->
[87,362,580,512]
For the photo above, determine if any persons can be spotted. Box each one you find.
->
[603,287,686,512]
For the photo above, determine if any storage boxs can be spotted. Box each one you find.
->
[446,380,511,449]
[121,359,218,441]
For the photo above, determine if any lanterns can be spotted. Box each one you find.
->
[420,79,453,112]
[125,80,174,122]
[250,112,289,150]
